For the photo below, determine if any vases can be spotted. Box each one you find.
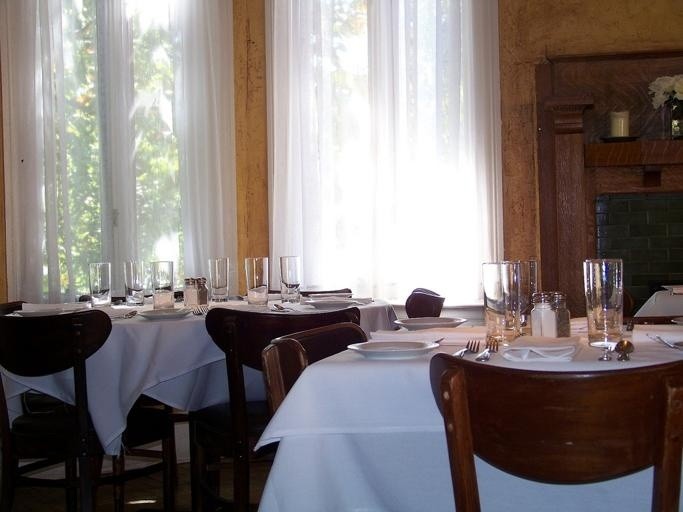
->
[670,108,682,141]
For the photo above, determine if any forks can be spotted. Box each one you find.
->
[190,305,208,315]
[474,341,499,362]
[646,332,683,350]
[452,339,480,359]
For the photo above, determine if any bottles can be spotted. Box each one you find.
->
[529,290,571,339]
[182,277,208,310]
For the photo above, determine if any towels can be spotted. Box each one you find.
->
[632,323,682,345]
[499,335,582,364]
[21,301,91,311]
[369,326,491,346]
[570,316,590,334]
[268,300,314,313]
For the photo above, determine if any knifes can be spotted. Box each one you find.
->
[598,345,612,362]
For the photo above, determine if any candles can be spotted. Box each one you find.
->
[607,111,630,137]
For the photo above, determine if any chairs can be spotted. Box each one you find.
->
[268,288,352,297]
[0,300,67,415]
[261,323,368,414]
[405,288,445,318]
[1,310,178,511]
[623,315,682,327]
[77,290,184,302]
[429,352,683,512]
[189,306,361,512]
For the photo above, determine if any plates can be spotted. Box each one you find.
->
[394,316,468,331]
[661,285,682,291]
[347,340,439,359]
[138,309,191,319]
[15,309,62,318]
[670,317,683,325]
[302,292,355,309]
[598,137,635,144]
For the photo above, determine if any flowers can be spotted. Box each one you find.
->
[647,74,682,110]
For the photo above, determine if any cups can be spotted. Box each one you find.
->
[242,256,269,306]
[279,255,300,305]
[480,257,538,346]
[581,258,625,348]
[208,257,230,303]
[610,110,628,137]
[87,259,174,311]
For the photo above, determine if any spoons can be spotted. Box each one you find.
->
[109,310,136,321]
[272,303,295,311]
[615,340,634,361]
